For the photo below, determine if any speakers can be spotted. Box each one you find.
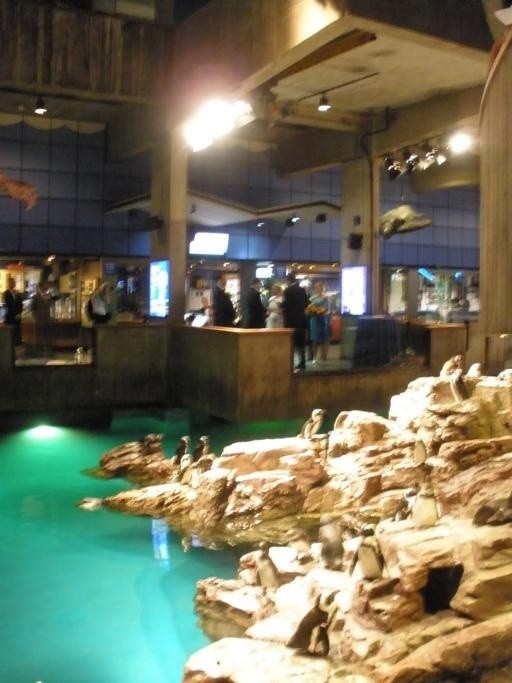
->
[349,232,363,249]
[317,213,326,223]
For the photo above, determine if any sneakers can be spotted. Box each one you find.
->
[295,355,326,369]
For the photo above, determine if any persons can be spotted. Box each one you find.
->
[2,270,334,371]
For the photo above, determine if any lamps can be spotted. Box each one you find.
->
[318,93,331,111]
[384,143,440,181]
